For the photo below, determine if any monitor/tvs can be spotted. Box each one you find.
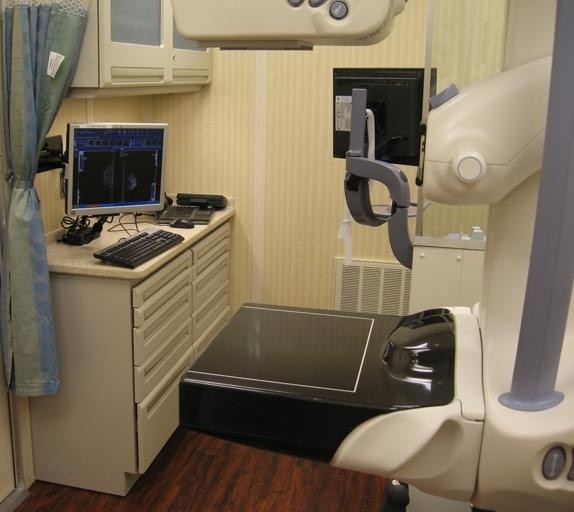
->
[62,122,169,215]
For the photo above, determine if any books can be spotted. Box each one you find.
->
[159,204,215,225]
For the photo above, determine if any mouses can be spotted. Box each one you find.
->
[169,217,195,228]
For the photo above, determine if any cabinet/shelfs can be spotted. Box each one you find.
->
[27,194,237,497]
[63,0,213,99]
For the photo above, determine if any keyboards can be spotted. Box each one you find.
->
[94,227,185,270]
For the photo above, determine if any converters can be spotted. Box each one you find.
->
[66,226,95,247]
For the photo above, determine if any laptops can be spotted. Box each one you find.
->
[155,192,214,224]
